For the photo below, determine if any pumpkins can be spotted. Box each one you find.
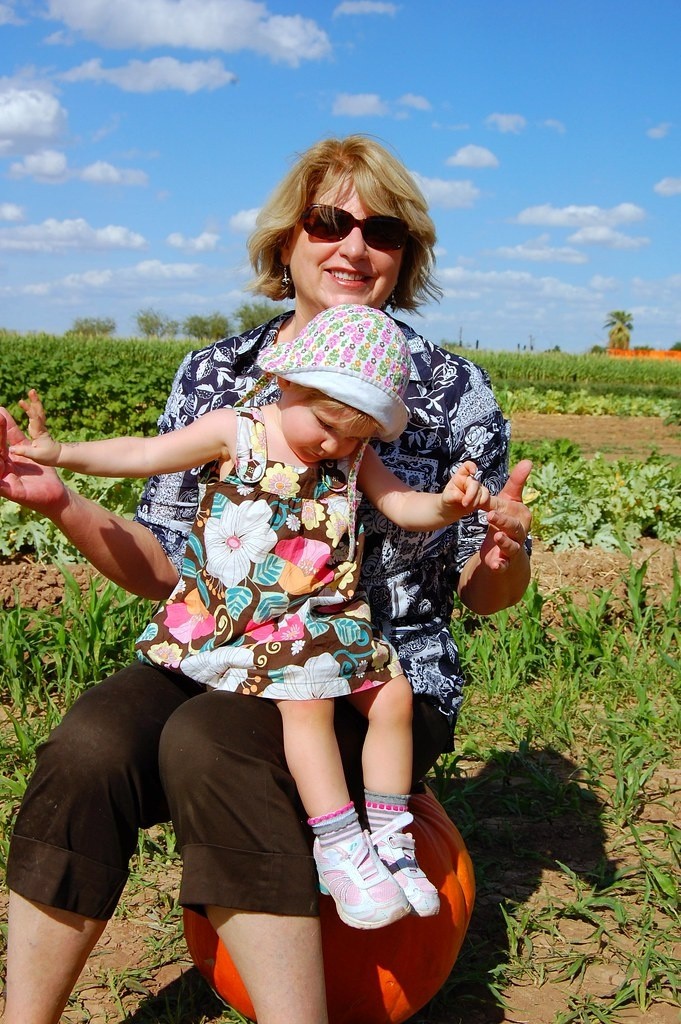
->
[183,779,476,1024]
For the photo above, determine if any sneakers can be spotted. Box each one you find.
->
[361,818,440,917]
[313,811,414,929]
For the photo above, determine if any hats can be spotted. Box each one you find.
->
[233,303,411,562]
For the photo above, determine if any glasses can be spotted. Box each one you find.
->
[301,203,409,250]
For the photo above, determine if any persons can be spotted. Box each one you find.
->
[0,138,534,1024]
[7,305,492,929]
